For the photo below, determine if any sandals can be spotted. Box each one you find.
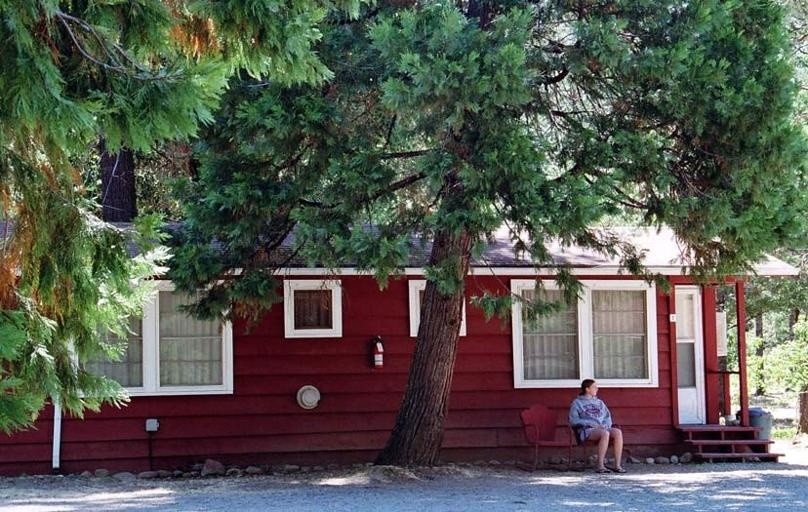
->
[595,466,611,473]
[614,466,627,472]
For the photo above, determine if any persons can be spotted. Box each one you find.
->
[567,378,627,473]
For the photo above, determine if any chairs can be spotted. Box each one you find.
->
[519,401,621,471]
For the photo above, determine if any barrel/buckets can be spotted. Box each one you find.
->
[749,413,773,440]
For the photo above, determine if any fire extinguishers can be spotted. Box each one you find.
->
[371,336,384,369]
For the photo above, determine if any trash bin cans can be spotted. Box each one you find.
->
[737,407,772,452]
